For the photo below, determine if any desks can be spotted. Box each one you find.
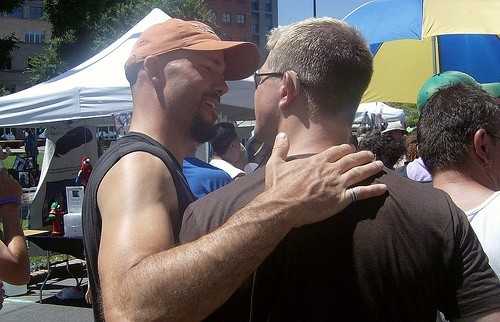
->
[21,230,86,305]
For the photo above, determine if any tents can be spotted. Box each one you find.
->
[354,102,405,133]
[0,9,256,165]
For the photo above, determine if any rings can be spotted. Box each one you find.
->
[349,187,358,202]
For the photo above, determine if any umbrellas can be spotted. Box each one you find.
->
[344,0,500,103]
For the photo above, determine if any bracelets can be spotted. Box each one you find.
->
[0,197,21,205]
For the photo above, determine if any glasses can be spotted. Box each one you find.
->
[254,71,308,89]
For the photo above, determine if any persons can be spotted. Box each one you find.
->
[0,20,500,322]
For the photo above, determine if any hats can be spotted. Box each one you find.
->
[124,18,262,81]
[246,130,255,149]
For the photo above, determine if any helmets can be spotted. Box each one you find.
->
[417,71,500,116]
